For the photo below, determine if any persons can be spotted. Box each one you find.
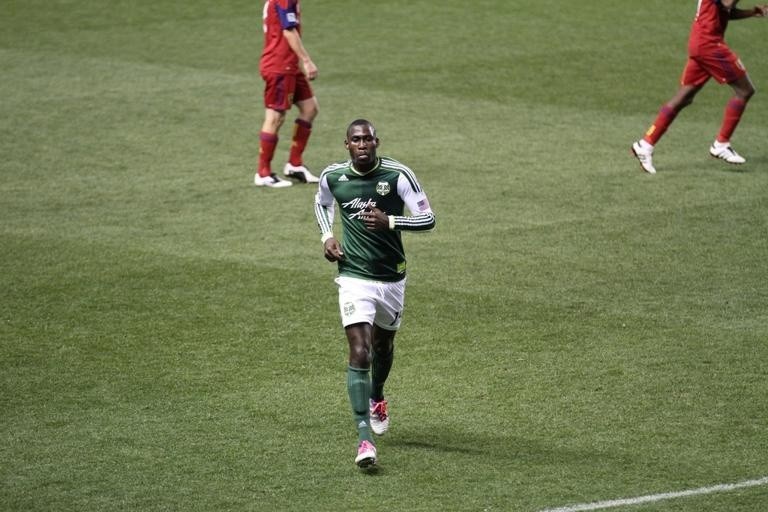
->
[632,0,767,176]
[315,120,435,467]
[254,1,320,187]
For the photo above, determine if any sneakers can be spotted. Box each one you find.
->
[283,163,320,183]
[369,399,389,436]
[254,173,292,188]
[709,140,746,163]
[355,441,377,469]
[631,139,658,174]
